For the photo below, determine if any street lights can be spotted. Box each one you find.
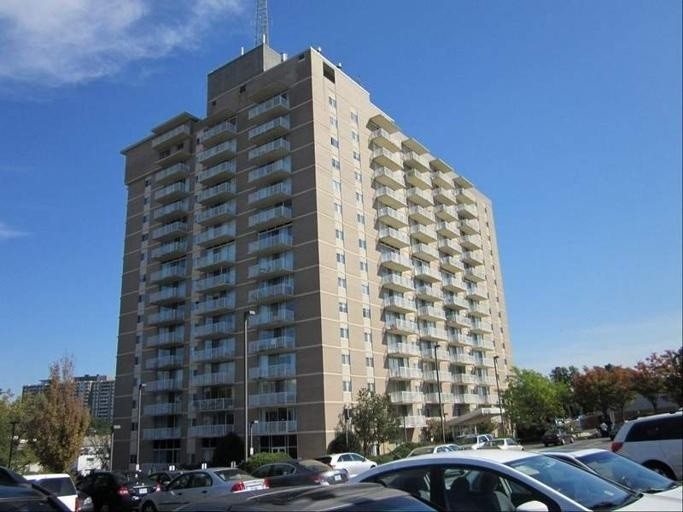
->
[492,355,506,437]
[241,310,255,458]
[134,383,146,479]
[433,344,448,442]
